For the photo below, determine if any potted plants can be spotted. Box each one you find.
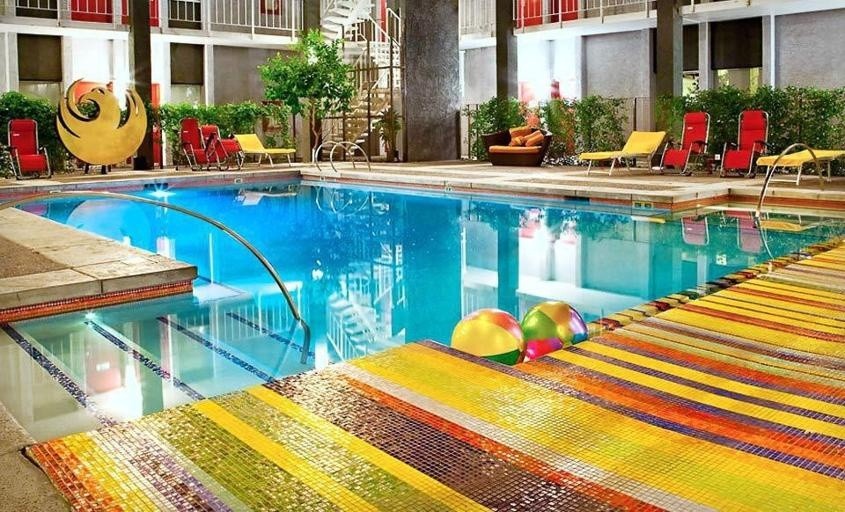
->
[376,108,402,162]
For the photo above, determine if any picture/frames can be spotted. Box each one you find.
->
[260,0,282,16]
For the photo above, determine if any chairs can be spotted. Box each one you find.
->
[4,120,297,181]
[658,111,710,175]
[481,127,553,167]
[720,110,769,179]
[678,211,764,253]
[233,185,299,206]
[756,148,844,186]
[579,130,666,177]
[19,199,53,219]
